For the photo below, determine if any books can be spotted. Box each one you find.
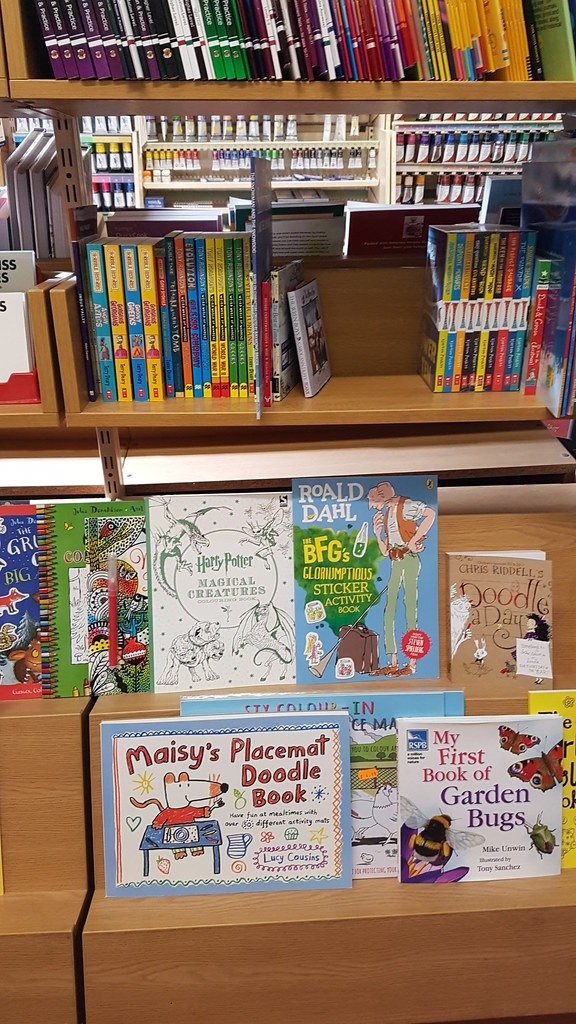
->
[444,549,555,689]
[480,176,525,225]
[346,200,480,269]
[1,475,576,899]
[1,198,344,406]
[36,0,575,82]
[419,224,576,419]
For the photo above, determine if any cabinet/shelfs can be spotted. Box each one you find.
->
[82,512,576,1024]
[1,696,90,1024]
[0,0,576,427]
[10,111,138,213]
[138,114,390,209]
[386,112,575,205]
